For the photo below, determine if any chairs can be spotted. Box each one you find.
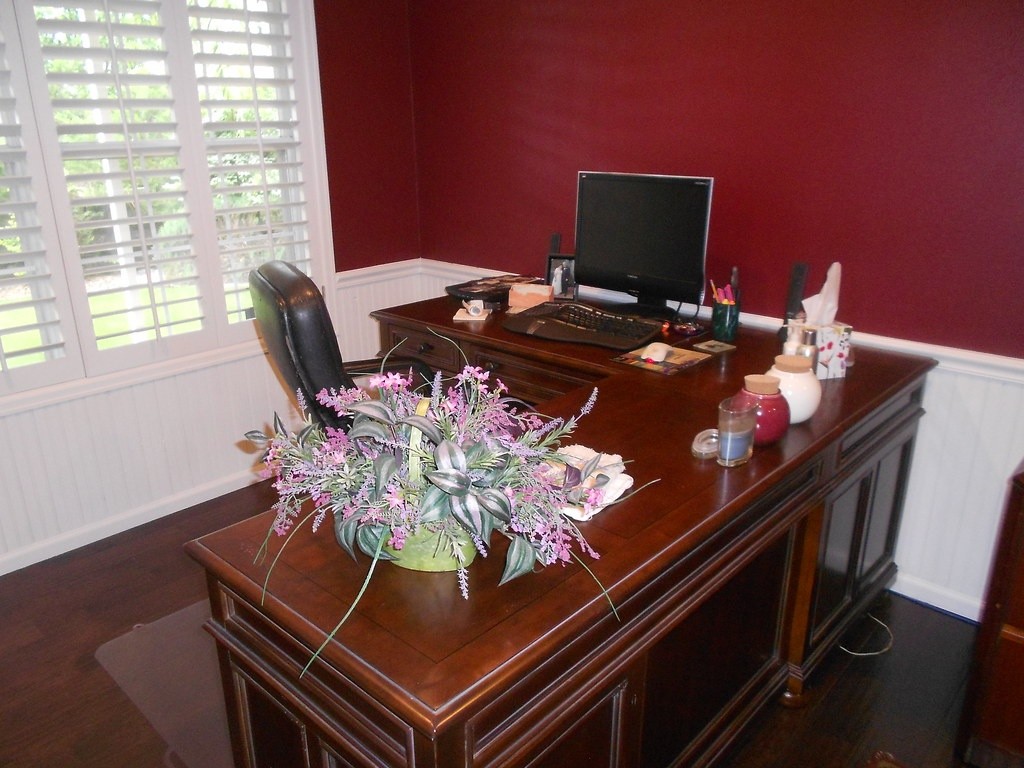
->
[951,458,1024,768]
[248,260,433,439]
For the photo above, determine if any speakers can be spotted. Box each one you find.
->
[549,231,562,253]
[779,262,810,341]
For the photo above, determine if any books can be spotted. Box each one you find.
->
[453,309,493,321]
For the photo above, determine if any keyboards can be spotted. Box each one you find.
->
[503,300,663,350]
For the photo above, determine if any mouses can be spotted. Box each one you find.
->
[641,343,674,363]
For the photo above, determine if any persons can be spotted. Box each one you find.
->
[551,261,571,296]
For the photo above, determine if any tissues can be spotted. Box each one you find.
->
[787,261,852,381]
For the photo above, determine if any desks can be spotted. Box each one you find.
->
[184,293,940,768]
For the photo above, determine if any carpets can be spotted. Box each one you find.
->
[95,599,236,768]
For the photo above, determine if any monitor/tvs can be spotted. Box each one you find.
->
[575,171,714,320]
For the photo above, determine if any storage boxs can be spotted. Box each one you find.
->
[509,284,554,307]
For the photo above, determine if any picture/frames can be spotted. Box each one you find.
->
[545,254,578,303]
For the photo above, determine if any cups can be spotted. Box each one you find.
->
[718,397,759,466]
[712,300,739,341]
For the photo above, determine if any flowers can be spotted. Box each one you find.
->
[244,327,661,679]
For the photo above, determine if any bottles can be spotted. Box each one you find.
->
[783,325,801,356]
[796,329,819,375]
